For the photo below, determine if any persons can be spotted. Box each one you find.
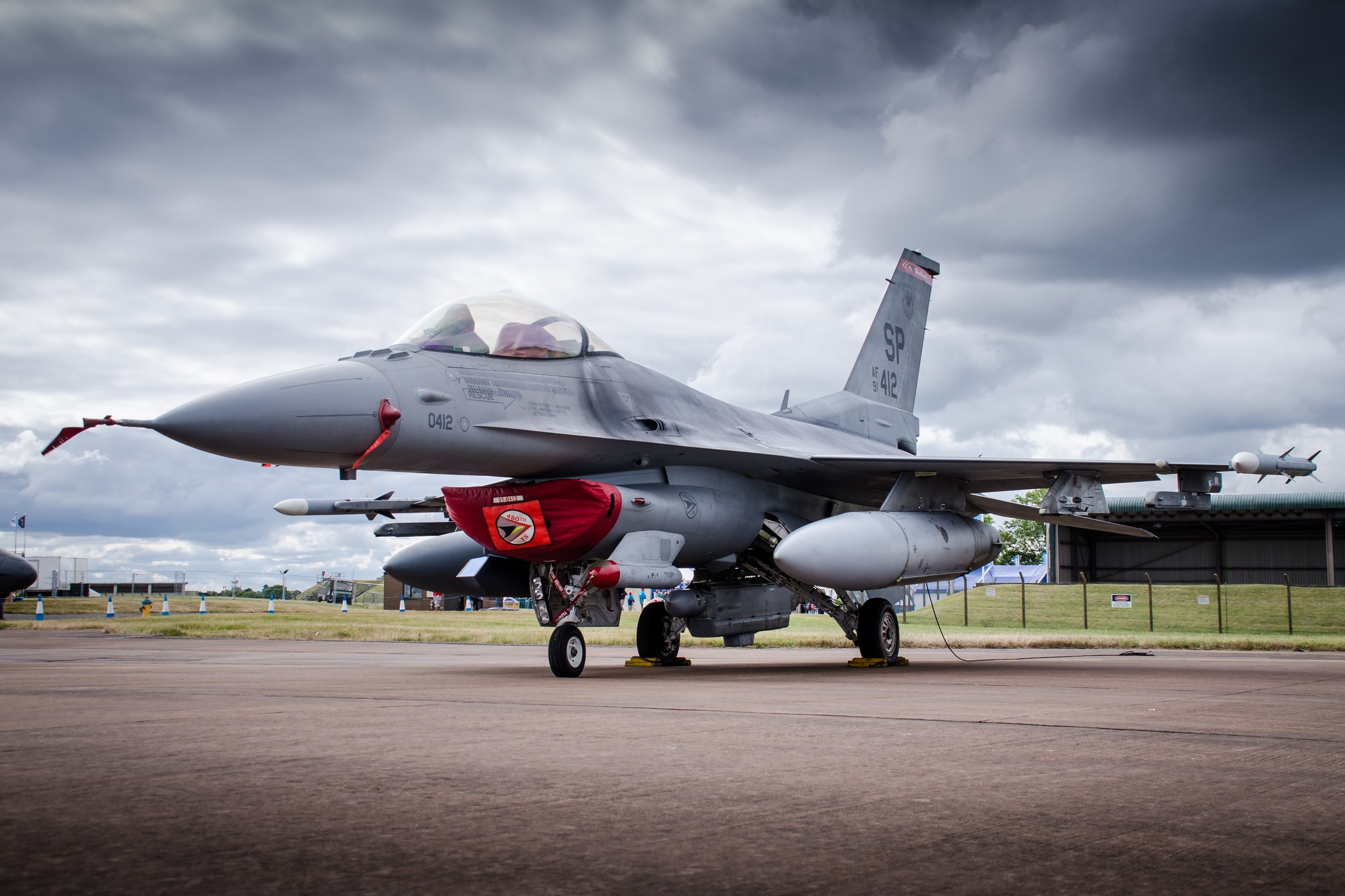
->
[627,592,635,611]
[620,590,628,611]
[655,587,677,599]
[639,588,645,611]
[433,592,444,611]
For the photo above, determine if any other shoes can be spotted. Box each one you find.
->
[440,607,443,611]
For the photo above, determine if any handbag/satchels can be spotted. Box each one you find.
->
[628,595,633,605]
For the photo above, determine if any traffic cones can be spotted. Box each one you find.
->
[342,595,348,612]
[162,594,169,616]
[268,594,275,614]
[35,594,45,620]
[465,596,471,611]
[106,595,114,619]
[200,594,206,614]
[400,595,406,612]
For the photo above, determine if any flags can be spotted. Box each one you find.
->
[11,516,17,527]
[18,515,26,528]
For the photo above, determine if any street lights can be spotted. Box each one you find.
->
[278,569,290,602]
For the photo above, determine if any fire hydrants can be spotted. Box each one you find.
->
[139,596,153,617]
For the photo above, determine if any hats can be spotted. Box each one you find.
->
[629,592,632,594]
[641,588,646,590]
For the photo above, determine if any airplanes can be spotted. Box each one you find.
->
[115,247,1322,679]
[0,549,37,592]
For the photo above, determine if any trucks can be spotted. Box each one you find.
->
[317,580,353,605]
